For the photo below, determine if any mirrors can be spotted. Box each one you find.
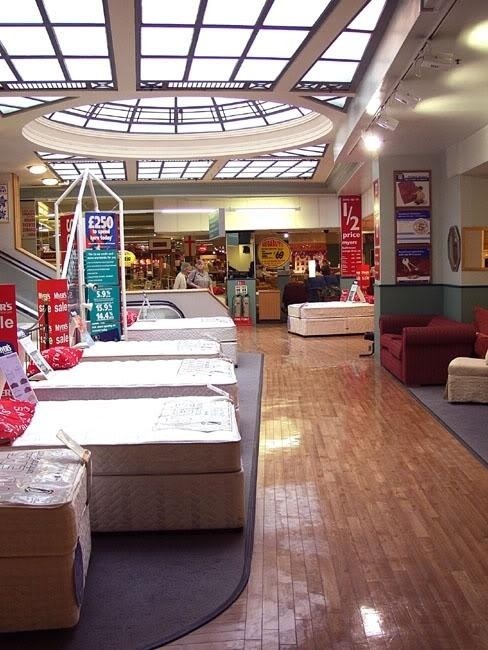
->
[462,227,488,272]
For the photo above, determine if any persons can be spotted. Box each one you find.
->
[203,255,225,268]
[187,259,214,296]
[133,257,160,282]
[172,263,191,290]
[414,186,425,203]
[281,259,338,314]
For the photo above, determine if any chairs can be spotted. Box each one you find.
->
[282,282,307,313]
[377,306,487,405]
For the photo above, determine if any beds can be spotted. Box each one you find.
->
[287,301,374,337]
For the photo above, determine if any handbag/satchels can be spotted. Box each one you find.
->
[187,271,198,289]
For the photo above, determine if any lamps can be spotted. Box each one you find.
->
[374,45,454,134]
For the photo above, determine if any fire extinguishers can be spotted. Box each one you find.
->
[232,294,250,317]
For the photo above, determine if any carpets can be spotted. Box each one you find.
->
[405,386,488,469]
[1,352,266,649]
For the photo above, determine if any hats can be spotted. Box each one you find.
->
[416,186,423,190]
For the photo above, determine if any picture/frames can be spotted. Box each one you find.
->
[392,169,432,286]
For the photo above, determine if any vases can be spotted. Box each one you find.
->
[445,225,462,273]
